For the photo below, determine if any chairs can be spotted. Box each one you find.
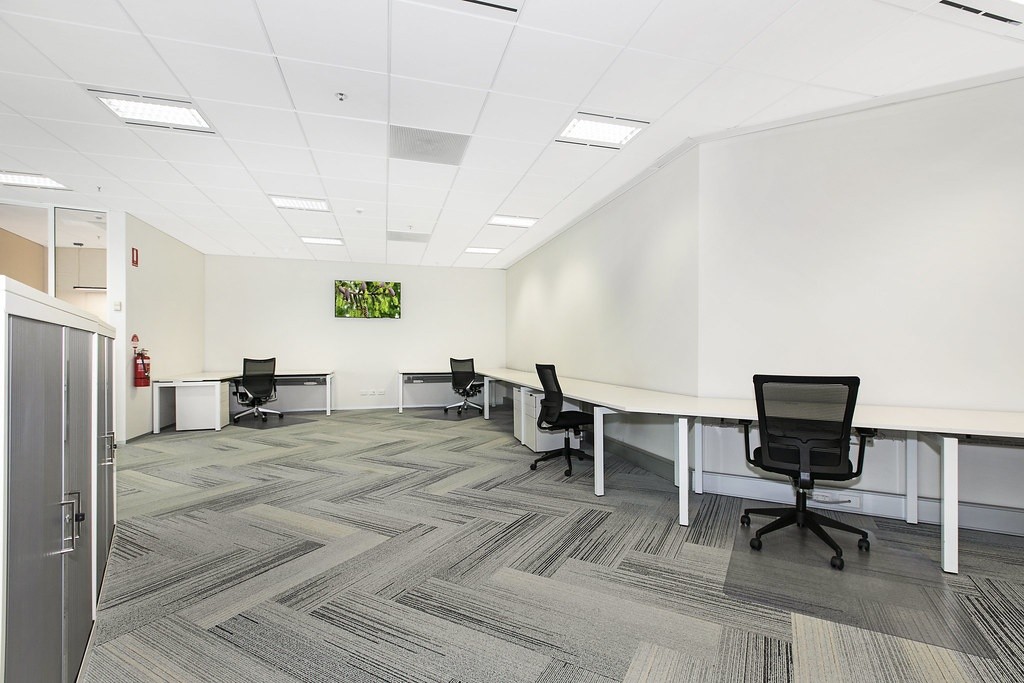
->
[232,357,284,426]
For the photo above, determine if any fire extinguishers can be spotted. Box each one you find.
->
[135,348,151,387]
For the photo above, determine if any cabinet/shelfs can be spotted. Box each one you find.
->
[174,381,231,431]
[1,274,93,683]
[95,318,118,619]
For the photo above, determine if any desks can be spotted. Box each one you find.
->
[151,371,335,435]
[398,363,1023,576]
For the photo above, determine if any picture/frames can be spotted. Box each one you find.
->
[334,280,401,319]
